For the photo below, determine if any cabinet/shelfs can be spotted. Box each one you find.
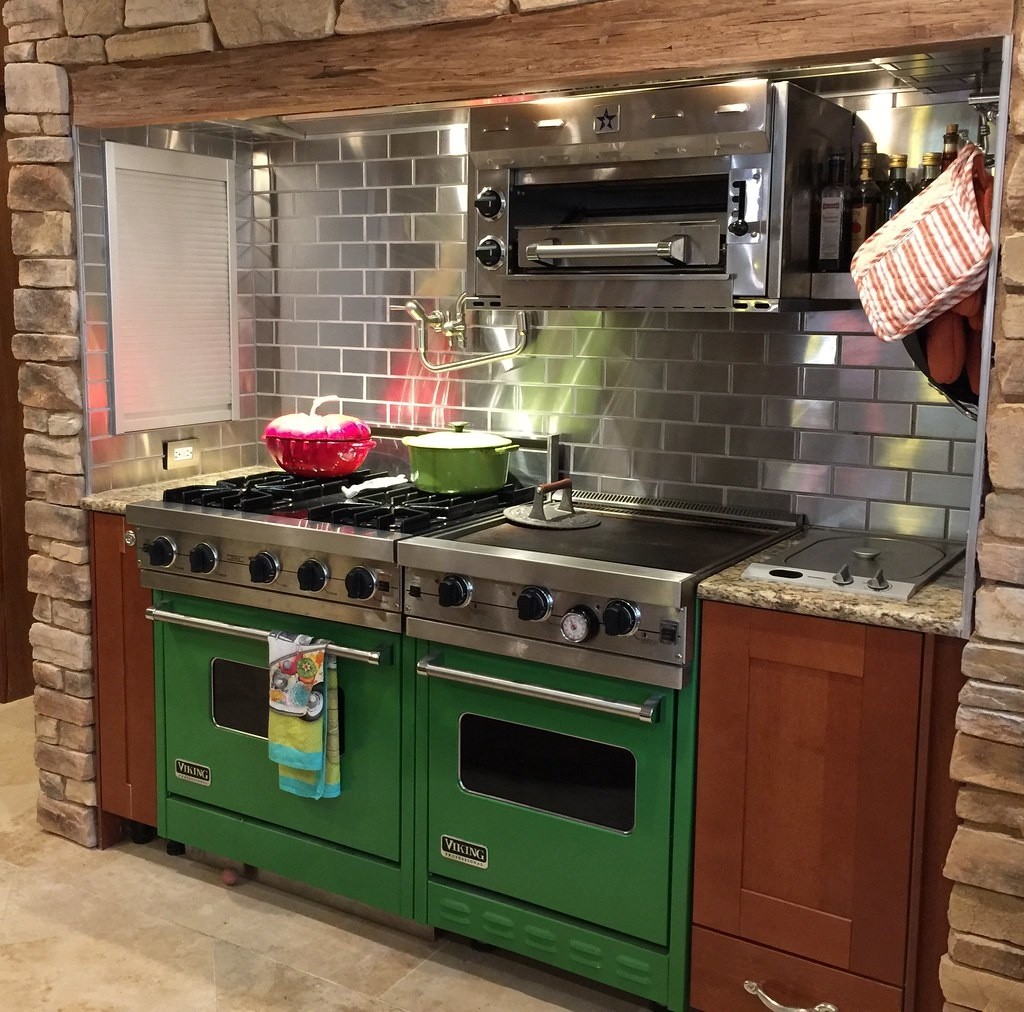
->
[688,599,971,1012]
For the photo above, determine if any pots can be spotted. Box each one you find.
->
[402,421,519,495]
[262,394,374,475]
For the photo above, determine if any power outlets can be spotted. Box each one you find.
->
[164,437,200,471]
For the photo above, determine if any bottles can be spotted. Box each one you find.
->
[814,122,977,274]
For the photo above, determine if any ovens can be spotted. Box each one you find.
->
[150,586,698,1012]
[461,71,854,316]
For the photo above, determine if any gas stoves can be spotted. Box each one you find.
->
[127,461,532,634]
